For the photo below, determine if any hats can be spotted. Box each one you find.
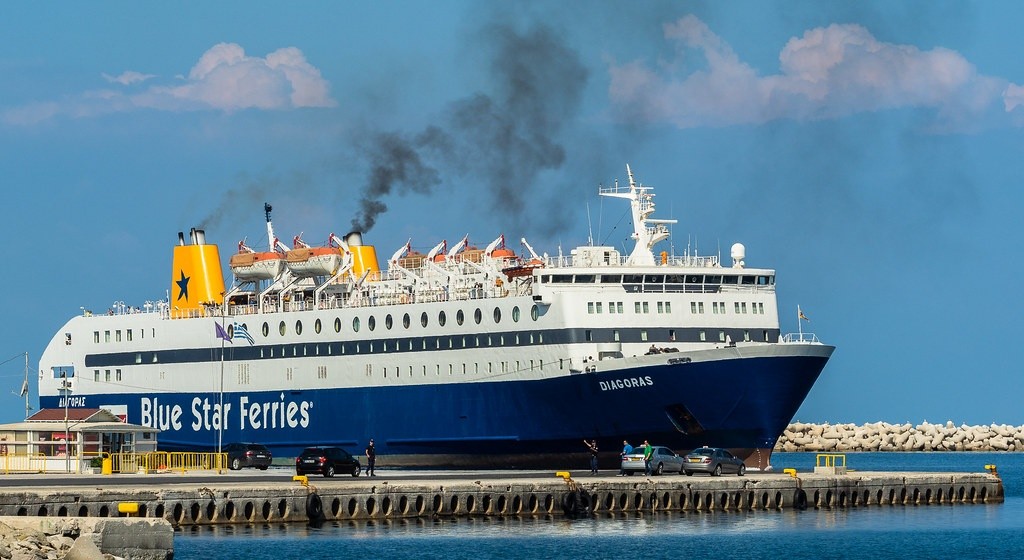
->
[592,440,595,443]
[370,439,374,442]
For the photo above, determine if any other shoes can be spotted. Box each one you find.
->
[365,472,368,475]
[617,474,623,476]
[371,474,376,476]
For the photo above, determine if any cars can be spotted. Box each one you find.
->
[682,446,747,477]
[621,446,685,476]
[202,443,273,471]
[294,446,362,477]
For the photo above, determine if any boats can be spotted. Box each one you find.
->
[227,250,284,280]
[281,245,340,276]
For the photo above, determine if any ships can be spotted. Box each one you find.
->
[36,162,836,477]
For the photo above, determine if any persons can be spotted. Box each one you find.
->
[264,293,360,312]
[365,439,376,476]
[643,439,653,476]
[443,275,504,302]
[616,440,633,476]
[584,440,598,475]
[85,306,141,316]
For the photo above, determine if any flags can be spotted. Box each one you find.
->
[798,307,810,323]
